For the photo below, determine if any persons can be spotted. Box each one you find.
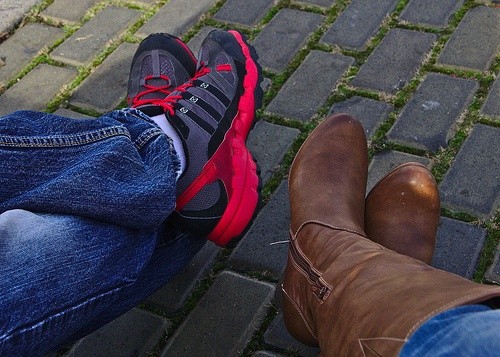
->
[273,113,500,357]
[0,28,263,357]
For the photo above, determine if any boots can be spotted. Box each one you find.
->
[274,112,500,357]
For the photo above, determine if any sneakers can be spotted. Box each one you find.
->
[126,28,264,248]
